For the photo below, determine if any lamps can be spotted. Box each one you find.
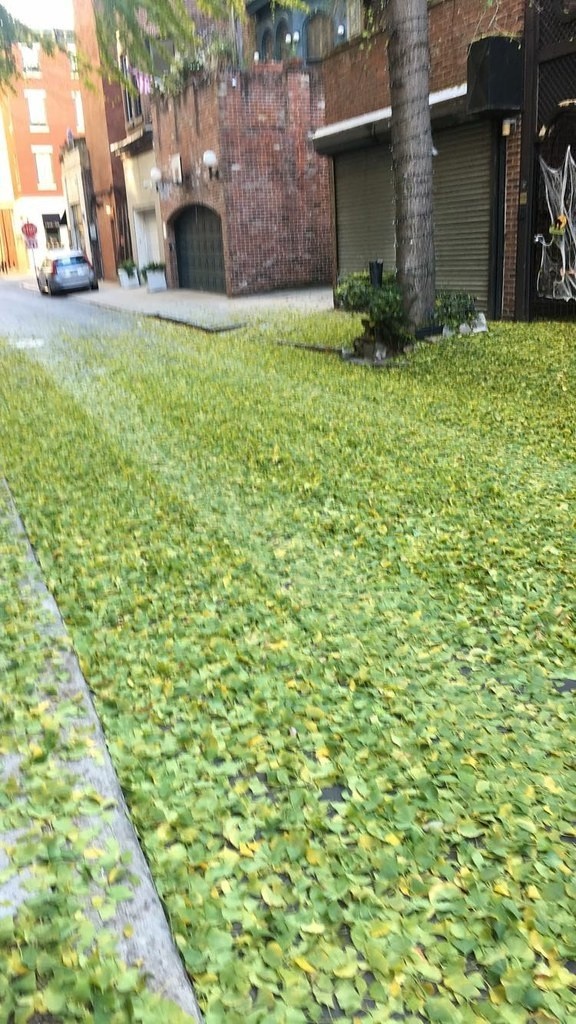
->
[151,167,165,193]
[203,149,219,180]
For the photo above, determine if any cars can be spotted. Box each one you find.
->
[37,251,100,298]
